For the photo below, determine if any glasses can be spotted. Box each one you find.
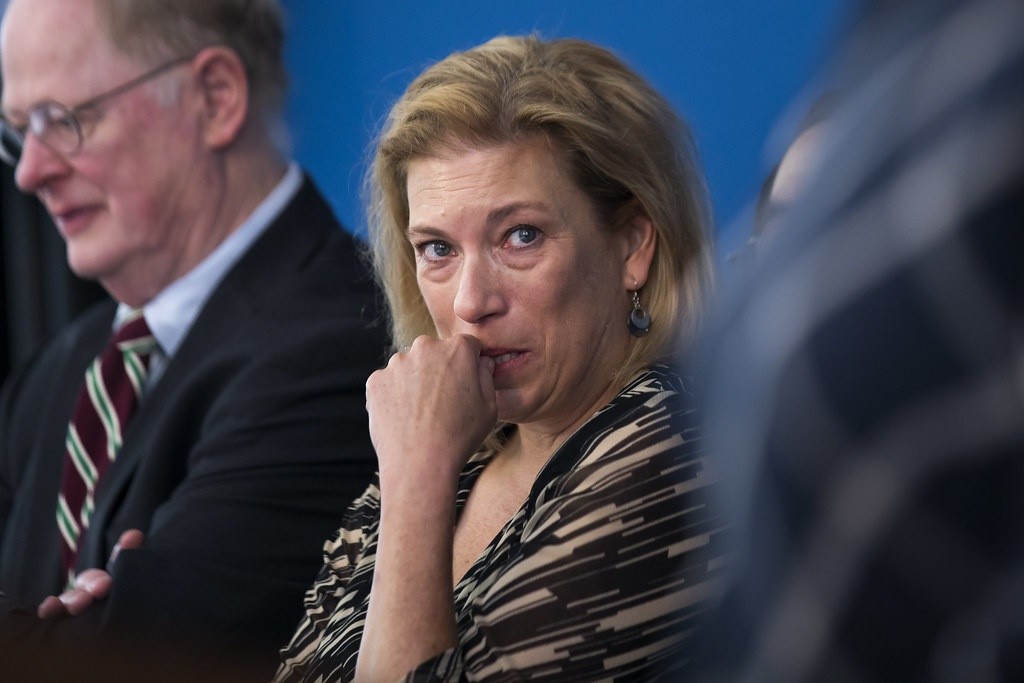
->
[1,55,197,172]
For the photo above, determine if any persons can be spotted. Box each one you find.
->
[0,0,396,683]
[277,31,733,683]
[741,94,847,277]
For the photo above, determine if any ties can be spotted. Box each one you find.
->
[45,303,156,606]
